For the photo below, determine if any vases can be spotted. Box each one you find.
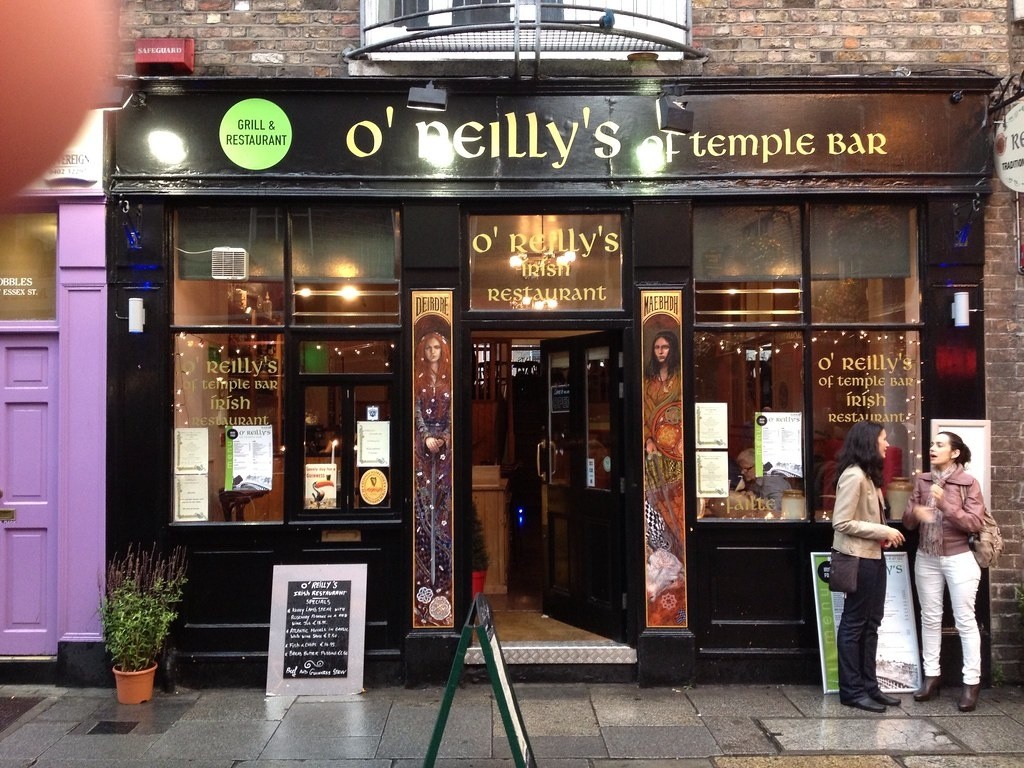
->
[709,491,752,519]
[887,477,915,520]
[782,490,805,517]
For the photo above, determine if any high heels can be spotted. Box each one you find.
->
[958,683,980,711]
[913,675,940,701]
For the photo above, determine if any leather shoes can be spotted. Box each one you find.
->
[847,697,885,713]
[868,689,901,706]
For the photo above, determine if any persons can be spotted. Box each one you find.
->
[736,448,792,511]
[832,420,906,713]
[902,431,984,712]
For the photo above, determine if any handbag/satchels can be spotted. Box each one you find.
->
[968,508,1002,567]
[828,552,859,593]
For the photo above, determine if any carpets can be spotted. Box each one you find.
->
[472,609,613,642]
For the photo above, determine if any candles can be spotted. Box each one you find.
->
[329,441,339,463]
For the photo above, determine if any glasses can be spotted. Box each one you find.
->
[739,465,755,473]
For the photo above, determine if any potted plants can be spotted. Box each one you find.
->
[472,502,490,602]
[95,544,189,706]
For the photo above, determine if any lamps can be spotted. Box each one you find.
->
[509,214,577,311]
[406,80,449,113]
[653,89,694,135]
[91,87,148,113]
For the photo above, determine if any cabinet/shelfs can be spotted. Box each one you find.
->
[715,332,800,427]
[229,314,283,452]
